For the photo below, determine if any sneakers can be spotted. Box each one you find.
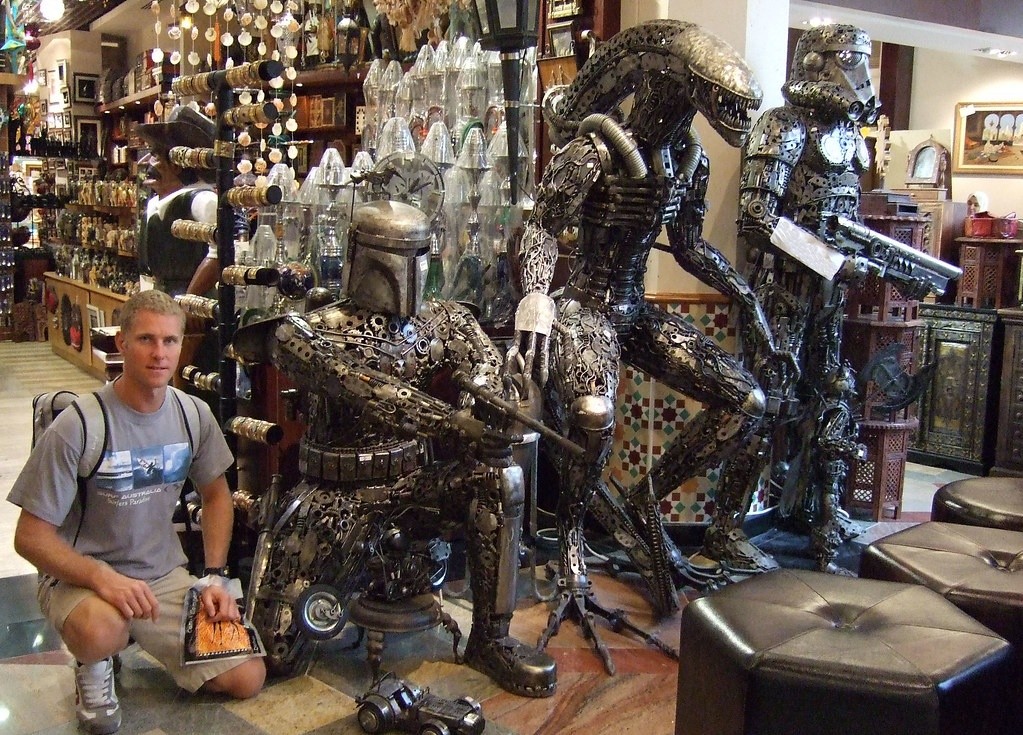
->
[73,655,122,734]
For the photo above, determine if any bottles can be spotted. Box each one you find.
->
[70,247,100,283]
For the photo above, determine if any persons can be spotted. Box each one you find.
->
[245,203,560,735]
[5,288,266,732]
[137,106,220,296]
[701,23,882,576]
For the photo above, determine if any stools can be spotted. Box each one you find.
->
[678,477,1023,735]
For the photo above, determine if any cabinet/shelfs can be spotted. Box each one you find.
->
[43,203,137,385]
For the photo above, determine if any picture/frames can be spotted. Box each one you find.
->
[63,111,72,129]
[951,102,1023,175]
[38,69,47,86]
[60,86,72,110]
[47,112,55,128]
[73,72,100,104]
[40,99,47,116]
[57,185,66,197]
[42,130,74,175]
[26,164,42,177]
[74,114,104,160]
[55,113,63,129]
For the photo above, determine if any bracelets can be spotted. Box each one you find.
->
[203,567,229,577]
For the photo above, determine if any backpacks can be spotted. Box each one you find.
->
[32,386,204,480]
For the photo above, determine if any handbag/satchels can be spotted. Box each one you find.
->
[178,572,268,667]
